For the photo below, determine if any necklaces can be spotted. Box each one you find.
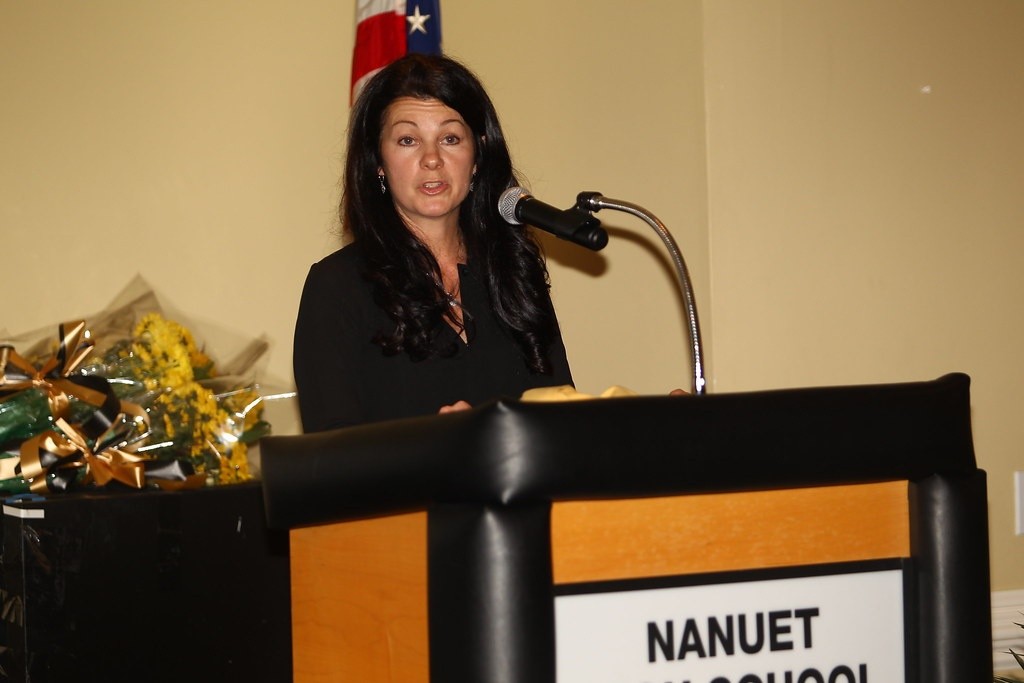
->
[428,246,463,306]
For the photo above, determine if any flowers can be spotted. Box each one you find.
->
[0,267,271,456]
[0,375,296,503]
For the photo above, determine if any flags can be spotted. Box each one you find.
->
[350,1,440,111]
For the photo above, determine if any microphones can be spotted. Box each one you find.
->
[498,187,608,252]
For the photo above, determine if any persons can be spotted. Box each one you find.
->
[291,55,576,434]
